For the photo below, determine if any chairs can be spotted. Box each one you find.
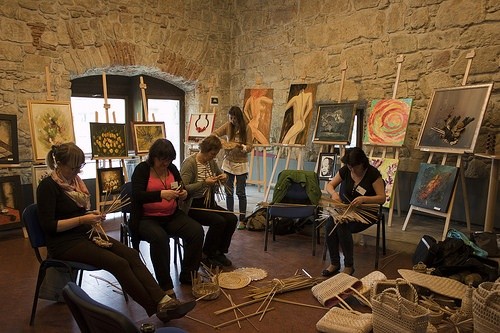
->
[323,178,387,270]
[262,169,322,257]
[21,203,129,326]
[118,182,205,265]
[62,281,187,333]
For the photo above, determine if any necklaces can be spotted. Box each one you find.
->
[152,166,171,201]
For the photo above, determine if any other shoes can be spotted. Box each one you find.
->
[157,299,193,318]
[321,264,340,276]
[237,224,244,230]
[179,272,203,284]
[349,268,355,275]
[207,253,231,266]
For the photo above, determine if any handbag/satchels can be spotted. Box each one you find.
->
[311,270,499,333]
[413,228,500,270]
[247,208,313,235]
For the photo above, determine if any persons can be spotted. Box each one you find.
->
[322,147,386,276]
[129,139,204,300]
[210,106,254,229]
[177,135,238,269]
[36,142,196,323]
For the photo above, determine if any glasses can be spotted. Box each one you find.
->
[68,162,86,171]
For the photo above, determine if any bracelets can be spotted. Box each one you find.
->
[331,192,336,197]
[78,216,81,226]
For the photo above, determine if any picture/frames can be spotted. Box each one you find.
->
[413,81,493,154]
[98,166,125,197]
[317,152,337,181]
[0,114,20,165]
[31,163,50,204]
[0,174,24,232]
[90,122,129,159]
[131,120,167,156]
[312,101,357,146]
[26,99,76,164]
